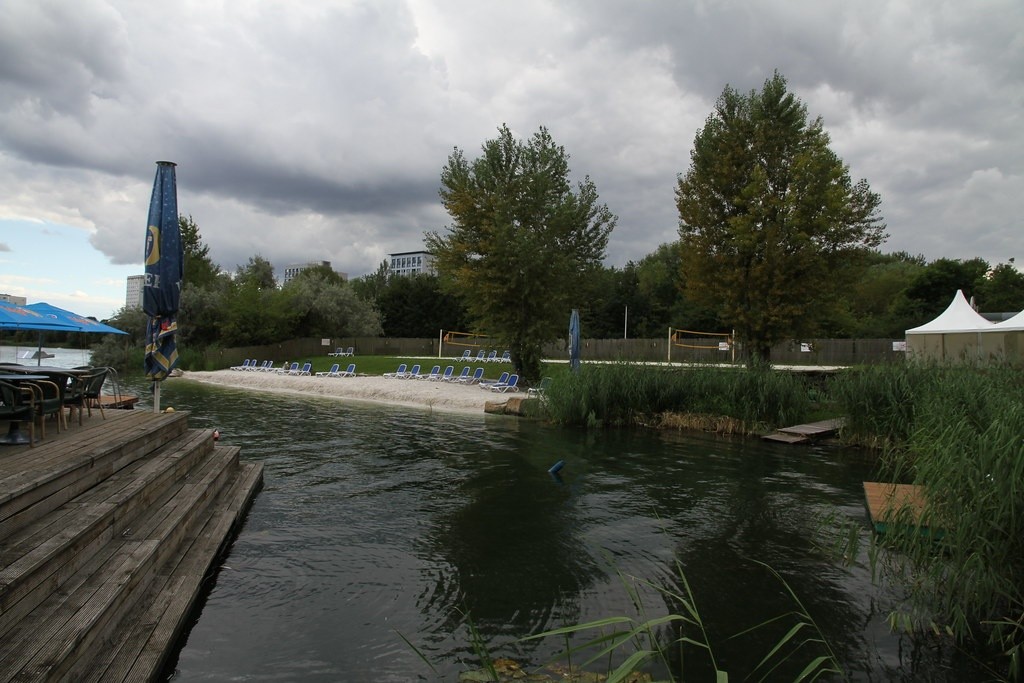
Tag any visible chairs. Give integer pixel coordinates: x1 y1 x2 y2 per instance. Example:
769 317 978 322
416 366 441 380
498 350 512 364
290 363 311 376
527 377 554 399
399 364 420 380
383 363 407 379
332 364 356 378
328 347 354 357
479 371 510 390
484 350 499 363
278 362 299 375
491 374 520 393
451 350 471 363
469 350 487 363
230 359 273 373
0 362 109 449
447 365 471 383
458 368 484 385
430 366 454 382
316 364 340 378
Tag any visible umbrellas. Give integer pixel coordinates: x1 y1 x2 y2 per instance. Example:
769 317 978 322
568 309 581 369
139 160 182 413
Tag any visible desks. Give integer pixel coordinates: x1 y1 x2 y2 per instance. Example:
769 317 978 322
0 374 50 445
0 366 90 426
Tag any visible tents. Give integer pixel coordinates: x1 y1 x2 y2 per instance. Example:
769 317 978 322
905 288 995 366
988 308 1024 366
